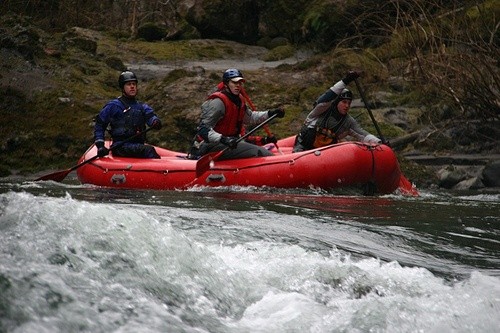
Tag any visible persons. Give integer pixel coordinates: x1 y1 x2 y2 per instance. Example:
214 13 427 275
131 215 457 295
292 71 389 153
94 71 162 158
190 68 285 160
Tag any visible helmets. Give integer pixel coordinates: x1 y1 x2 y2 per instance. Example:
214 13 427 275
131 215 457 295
338 87 353 99
119 71 137 88
223 69 244 85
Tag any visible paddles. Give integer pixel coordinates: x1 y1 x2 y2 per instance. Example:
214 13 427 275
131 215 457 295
36 125 154 182
352 73 419 197
196 113 276 178
241 88 283 155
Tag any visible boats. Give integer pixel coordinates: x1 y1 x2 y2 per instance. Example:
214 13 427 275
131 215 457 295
76 131 403 198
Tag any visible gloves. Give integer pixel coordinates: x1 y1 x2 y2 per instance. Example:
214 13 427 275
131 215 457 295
220 136 237 149
97 147 109 157
346 69 359 82
268 107 285 118
152 119 161 130
